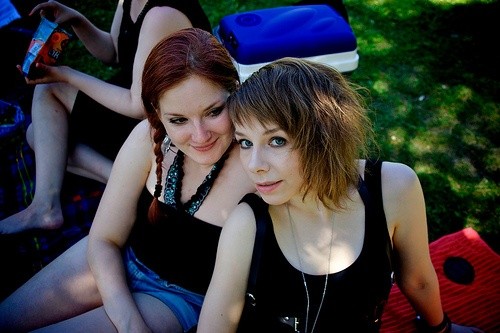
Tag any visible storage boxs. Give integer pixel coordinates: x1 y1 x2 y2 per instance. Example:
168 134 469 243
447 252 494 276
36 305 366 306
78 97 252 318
211 4 359 86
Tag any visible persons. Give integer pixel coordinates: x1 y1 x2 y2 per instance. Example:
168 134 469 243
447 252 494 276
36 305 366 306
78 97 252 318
0 28 257 333
0 0 213 235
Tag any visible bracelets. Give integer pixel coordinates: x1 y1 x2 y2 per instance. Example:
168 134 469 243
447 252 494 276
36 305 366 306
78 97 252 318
424 311 448 333
443 318 451 333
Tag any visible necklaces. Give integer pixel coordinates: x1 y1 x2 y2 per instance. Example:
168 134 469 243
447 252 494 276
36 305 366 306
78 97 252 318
196 56 482 333
282 200 338 333
